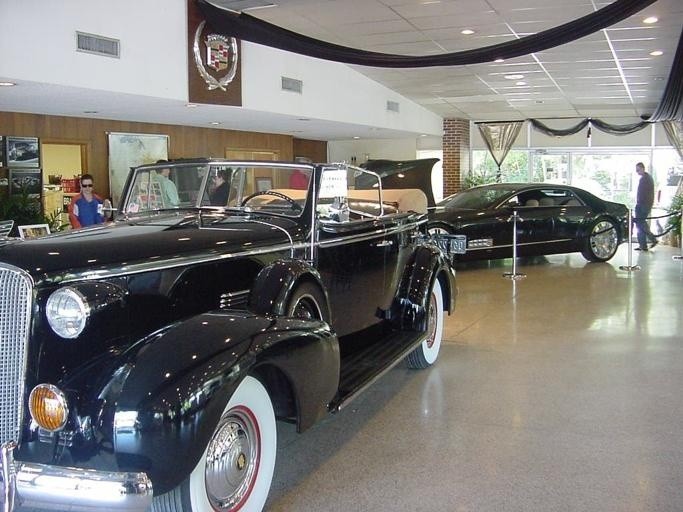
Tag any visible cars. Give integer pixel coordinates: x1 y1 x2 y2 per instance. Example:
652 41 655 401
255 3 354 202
8 143 39 162
0 154 459 510
416 180 635 266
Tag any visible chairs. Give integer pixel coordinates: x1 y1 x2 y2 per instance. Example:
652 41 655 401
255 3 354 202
540 196 553 205
525 196 539 206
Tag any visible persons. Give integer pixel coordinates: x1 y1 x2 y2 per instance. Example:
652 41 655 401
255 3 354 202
67 174 107 230
208 168 236 205
632 161 657 251
146 159 180 210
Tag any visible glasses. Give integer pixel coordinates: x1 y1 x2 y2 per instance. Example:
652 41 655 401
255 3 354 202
81 183 94 187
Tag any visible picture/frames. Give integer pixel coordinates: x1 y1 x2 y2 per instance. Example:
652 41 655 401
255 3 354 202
17 222 54 241
255 175 273 194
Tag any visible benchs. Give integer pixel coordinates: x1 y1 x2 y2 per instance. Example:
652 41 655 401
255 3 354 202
227 186 431 223
